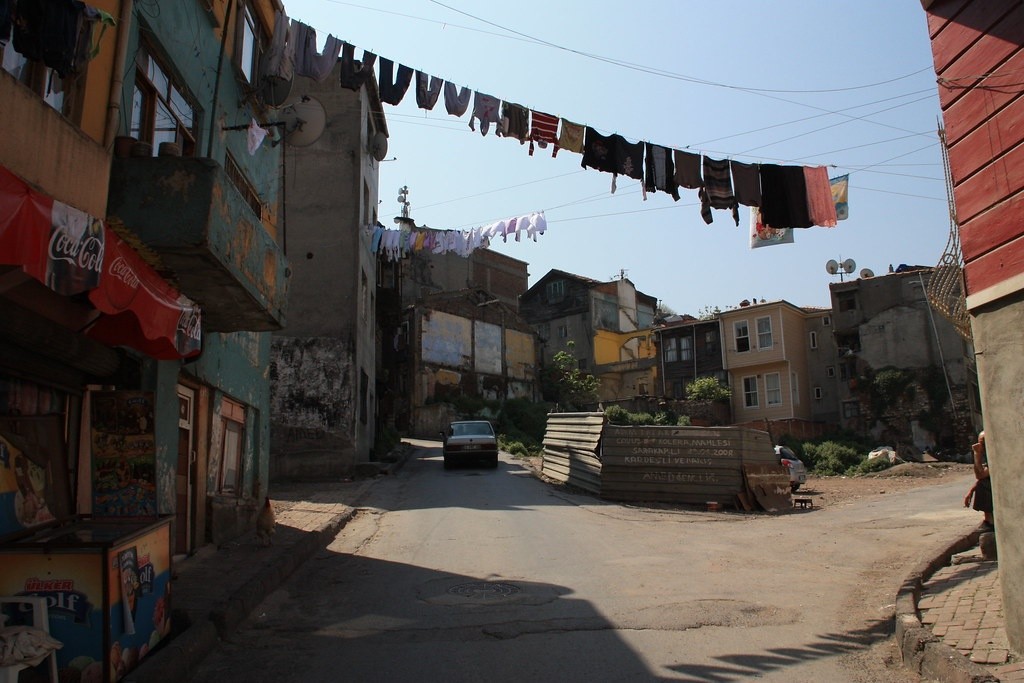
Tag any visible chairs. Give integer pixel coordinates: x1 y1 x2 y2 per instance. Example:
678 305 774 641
0 595 60 683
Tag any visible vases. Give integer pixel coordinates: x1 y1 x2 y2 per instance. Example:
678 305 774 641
553 340 602 412
114 136 179 159
681 375 731 426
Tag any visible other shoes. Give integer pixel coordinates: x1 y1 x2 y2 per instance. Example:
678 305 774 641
973 520 993 533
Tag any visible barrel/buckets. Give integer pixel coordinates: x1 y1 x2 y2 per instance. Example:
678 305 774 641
706 502 718 511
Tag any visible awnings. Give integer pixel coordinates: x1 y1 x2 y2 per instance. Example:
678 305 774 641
0 165 200 361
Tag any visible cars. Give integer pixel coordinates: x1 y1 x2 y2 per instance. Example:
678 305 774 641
439 421 499 470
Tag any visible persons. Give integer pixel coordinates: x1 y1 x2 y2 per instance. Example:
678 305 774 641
964 430 994 529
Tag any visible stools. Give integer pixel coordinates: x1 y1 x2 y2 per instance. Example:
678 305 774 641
795 499 812 509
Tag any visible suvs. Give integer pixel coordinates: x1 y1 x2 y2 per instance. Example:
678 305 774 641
774 446 806 491
